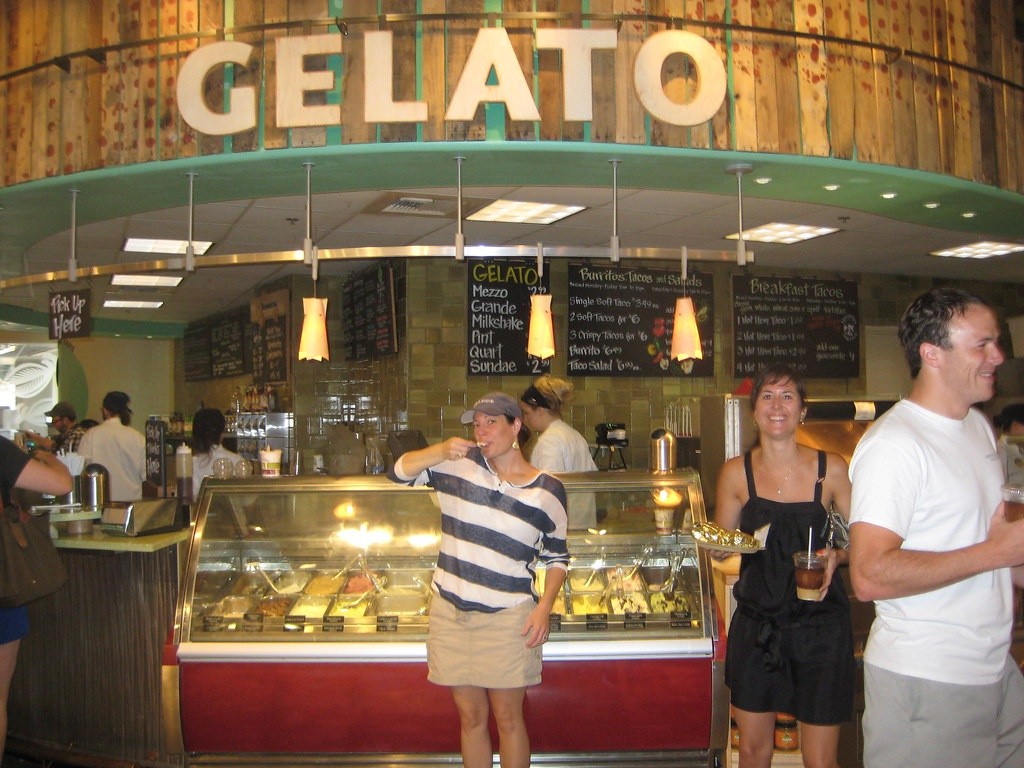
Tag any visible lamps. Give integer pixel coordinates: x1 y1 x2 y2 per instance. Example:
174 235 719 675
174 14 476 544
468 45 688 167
67 159 748 360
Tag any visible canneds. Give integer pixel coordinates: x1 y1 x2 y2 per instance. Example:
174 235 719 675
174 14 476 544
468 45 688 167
774 713 800 752
731 714 740 750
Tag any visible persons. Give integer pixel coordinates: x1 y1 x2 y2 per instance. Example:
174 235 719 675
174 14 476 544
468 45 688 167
172 409 245 496
387 391 570 767
0 434 73 764
22 401 97 453
520 376 598 532
78 391 147 501
847 289 1024 768
992 403 1024 486
707 367 853 768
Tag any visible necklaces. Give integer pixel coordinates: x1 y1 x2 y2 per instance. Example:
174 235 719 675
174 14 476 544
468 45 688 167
768 467 790 494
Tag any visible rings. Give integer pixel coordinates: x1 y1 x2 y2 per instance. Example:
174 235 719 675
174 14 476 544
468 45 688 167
722 555 726 559
458 455 463 458
543 637 546 639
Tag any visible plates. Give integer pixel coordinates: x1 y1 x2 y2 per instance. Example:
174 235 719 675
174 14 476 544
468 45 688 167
690 538 759 553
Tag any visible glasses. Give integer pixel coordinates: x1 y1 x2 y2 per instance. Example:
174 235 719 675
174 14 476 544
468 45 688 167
52 419 62 424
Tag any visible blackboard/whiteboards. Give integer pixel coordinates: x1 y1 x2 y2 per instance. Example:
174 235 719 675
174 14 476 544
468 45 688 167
145 423 163 485
731 275 860 380
251 321 289 383
341 267 395 360
566 264 714 379
48 289 90 339
467 259 550 377
181 314 245 379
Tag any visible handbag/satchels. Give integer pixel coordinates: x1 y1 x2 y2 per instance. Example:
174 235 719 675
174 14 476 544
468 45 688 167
820 510 850 552
0 489 72 609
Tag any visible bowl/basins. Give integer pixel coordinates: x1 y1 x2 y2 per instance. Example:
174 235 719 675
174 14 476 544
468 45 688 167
211 458 253 477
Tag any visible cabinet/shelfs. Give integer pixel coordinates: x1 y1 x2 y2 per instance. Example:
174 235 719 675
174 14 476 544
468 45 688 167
237 412 290 462
164 469 715 662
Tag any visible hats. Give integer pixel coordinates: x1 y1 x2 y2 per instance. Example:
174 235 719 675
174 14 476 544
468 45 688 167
44 401 77 418
460 391 523 425
102 391 132 413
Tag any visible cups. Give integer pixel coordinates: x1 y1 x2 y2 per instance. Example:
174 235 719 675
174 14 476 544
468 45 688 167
259 451 281 478
793 550 828 601
1000 483 1024 522
56 475 81 504
653 506 674 535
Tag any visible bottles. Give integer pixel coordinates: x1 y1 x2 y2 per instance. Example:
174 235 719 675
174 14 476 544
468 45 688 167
40 436 56 505
231 384 277 412
161 412 193 437
224 416 237 433
176 442 193 498
